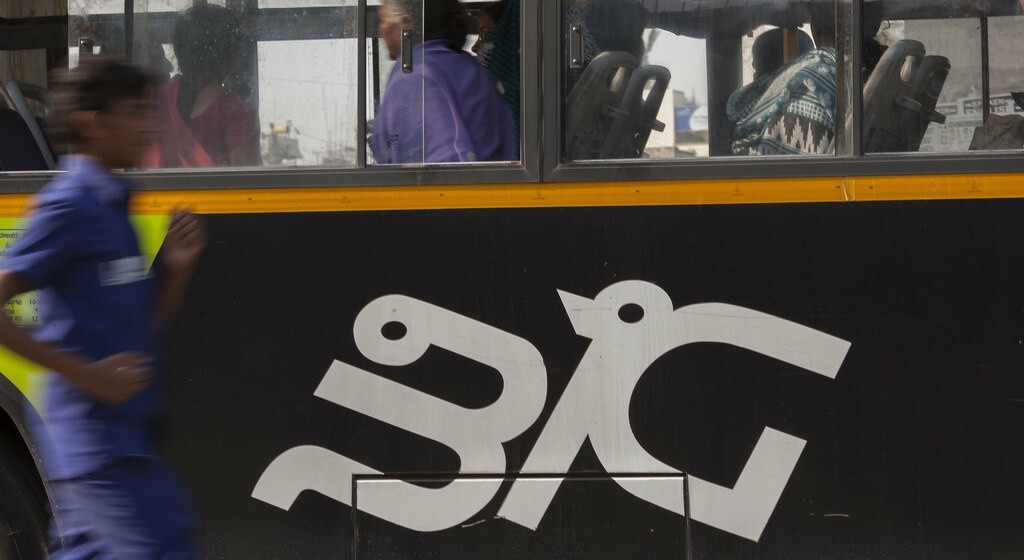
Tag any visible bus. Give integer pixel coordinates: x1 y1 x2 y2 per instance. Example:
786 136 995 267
2 0 1024 559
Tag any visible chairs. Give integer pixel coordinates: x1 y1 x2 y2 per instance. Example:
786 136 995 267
825 39 952 155
565 51 670 161
2 78 59 169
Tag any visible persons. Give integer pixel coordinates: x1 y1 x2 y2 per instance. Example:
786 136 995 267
0 56 207 560
135 6 260 169
368 0 517 163
724 0 890 159
